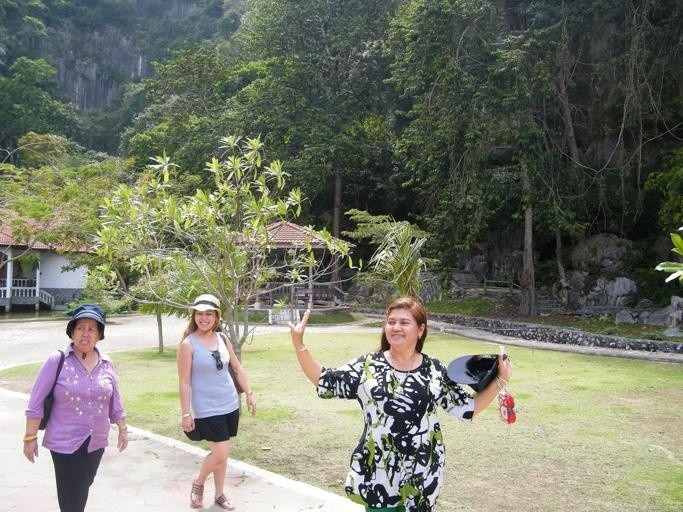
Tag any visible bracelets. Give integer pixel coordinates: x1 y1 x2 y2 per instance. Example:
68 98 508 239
296 346 308 353
246 389 252 395
182 413 191 419
23 435 37 443
496 377 503 391
120 426 127 430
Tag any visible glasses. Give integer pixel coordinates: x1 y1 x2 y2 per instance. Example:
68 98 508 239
210 349 224 371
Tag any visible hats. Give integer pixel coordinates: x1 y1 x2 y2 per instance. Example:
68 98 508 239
188 291 222 317
443 350 508 394
64 303 107 341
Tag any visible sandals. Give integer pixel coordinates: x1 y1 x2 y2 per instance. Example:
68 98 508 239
188 477 205 509
212 492 236 511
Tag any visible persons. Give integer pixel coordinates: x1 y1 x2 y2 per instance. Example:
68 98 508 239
23 304 128 512
176 294 257 511
287 296 513 512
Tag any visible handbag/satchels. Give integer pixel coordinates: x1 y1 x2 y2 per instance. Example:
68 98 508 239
35 346 68 432
215 331 246 393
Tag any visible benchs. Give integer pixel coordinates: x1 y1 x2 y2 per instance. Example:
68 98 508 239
292 285 333 306
244 287 273 306
